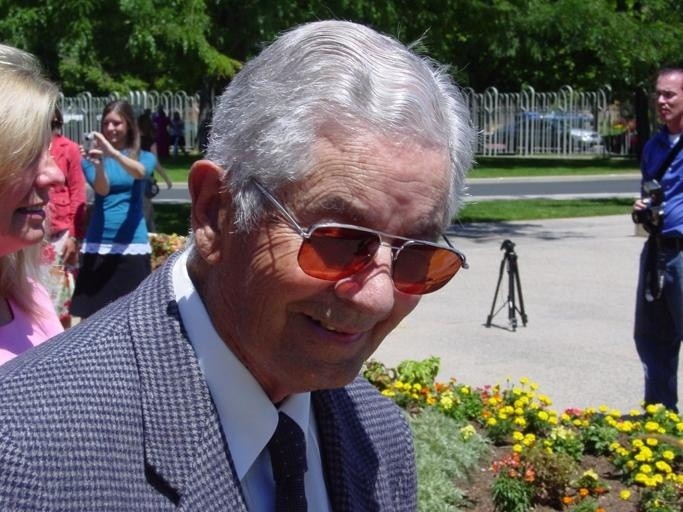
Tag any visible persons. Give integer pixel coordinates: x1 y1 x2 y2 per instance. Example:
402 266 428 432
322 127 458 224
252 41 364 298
0 43 64 370
632 62 681 417
135 103 212 164
135 113 173 235
71 99 155 318
0 14 478 510
36 104 86 329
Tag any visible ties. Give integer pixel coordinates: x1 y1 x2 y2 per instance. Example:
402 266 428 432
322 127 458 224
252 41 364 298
266 412 307 512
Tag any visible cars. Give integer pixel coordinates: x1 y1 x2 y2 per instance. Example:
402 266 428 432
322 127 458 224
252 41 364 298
479 108 612 159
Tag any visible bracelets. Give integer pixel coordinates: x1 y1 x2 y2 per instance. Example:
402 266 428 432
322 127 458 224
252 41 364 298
70 236 78 244
111 150 120 160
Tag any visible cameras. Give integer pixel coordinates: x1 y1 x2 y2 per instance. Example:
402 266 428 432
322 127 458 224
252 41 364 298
632 179 665 234
82 132 95 151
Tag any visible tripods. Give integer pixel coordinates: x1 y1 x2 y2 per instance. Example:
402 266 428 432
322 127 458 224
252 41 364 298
487 240 527 330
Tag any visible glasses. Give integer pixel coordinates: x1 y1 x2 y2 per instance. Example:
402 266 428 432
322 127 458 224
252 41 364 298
251 178 468 294
